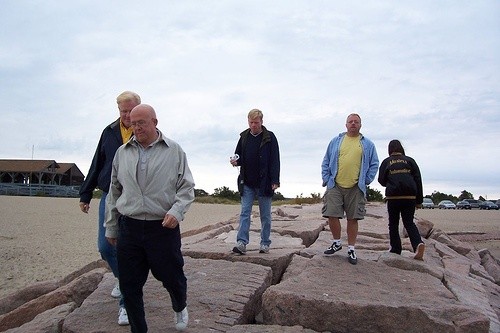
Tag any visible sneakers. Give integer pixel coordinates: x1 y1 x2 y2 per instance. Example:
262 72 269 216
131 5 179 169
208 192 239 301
175 306 188 330
347 249 357 265
259 244 270 253
232 240 247 255
112 281 121 297
324 241 342 256
116 308 129 325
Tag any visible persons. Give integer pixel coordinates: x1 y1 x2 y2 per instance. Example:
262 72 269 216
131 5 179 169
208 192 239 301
103 103 195 333
230 109 281 255
377 140 425 261
78 90 142 326
321 113 379 264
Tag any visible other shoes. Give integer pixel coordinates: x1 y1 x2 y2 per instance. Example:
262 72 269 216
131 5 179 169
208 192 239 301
414 243 425 258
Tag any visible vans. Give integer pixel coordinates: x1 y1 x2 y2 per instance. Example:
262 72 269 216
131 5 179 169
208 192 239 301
463 199 483 208
417 198 434 209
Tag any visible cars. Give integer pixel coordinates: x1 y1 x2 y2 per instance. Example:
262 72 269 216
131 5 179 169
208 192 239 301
438 200 456 210
457 201 472 210
479 199 500 210
